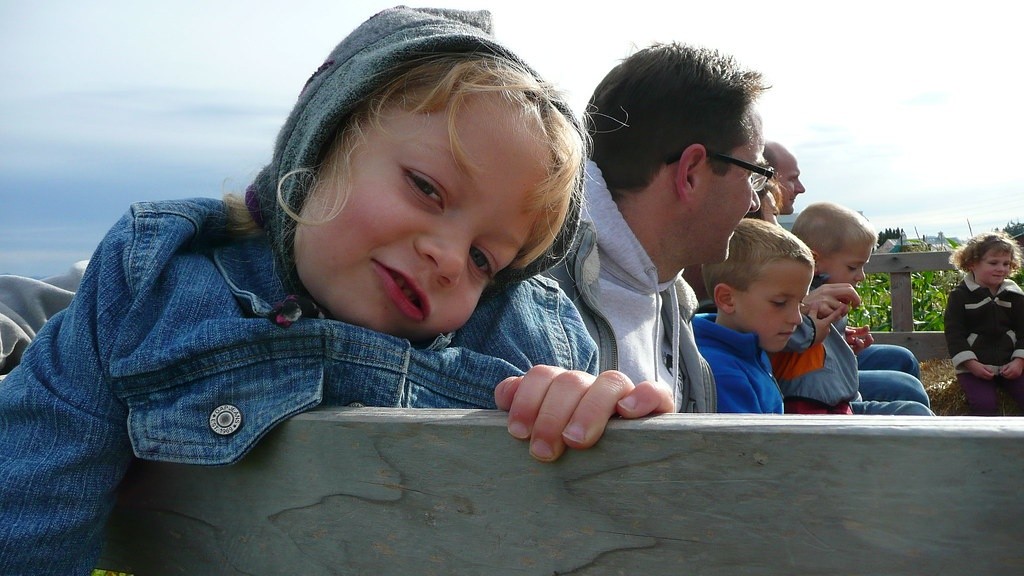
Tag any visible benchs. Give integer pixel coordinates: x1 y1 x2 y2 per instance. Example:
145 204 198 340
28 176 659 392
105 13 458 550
88 403 1024 576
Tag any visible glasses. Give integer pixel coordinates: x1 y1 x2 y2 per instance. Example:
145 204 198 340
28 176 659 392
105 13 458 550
660 149 773 191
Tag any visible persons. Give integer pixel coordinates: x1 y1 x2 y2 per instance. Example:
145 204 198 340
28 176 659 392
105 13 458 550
0 4 1023 576
